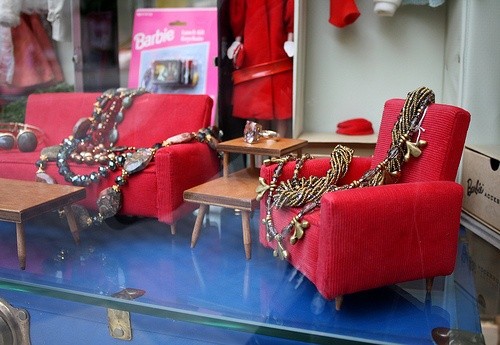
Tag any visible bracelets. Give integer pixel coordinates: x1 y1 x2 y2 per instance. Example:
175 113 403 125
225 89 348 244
0 120 45 152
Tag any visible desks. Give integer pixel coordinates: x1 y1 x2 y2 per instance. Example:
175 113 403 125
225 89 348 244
215 134 308 181
0 176 87 271
183 165 265 262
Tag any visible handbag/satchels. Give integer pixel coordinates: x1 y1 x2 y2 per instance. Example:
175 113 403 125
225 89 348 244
226 41 245 69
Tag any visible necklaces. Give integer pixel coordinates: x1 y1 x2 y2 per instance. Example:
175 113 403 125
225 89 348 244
255 86 436 261
36 88 227 229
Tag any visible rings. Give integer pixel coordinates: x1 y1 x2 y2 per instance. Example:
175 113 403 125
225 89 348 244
243 119 286 144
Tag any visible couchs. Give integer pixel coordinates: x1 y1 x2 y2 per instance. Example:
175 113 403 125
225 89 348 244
0 93 220 236
258 98 472 312
